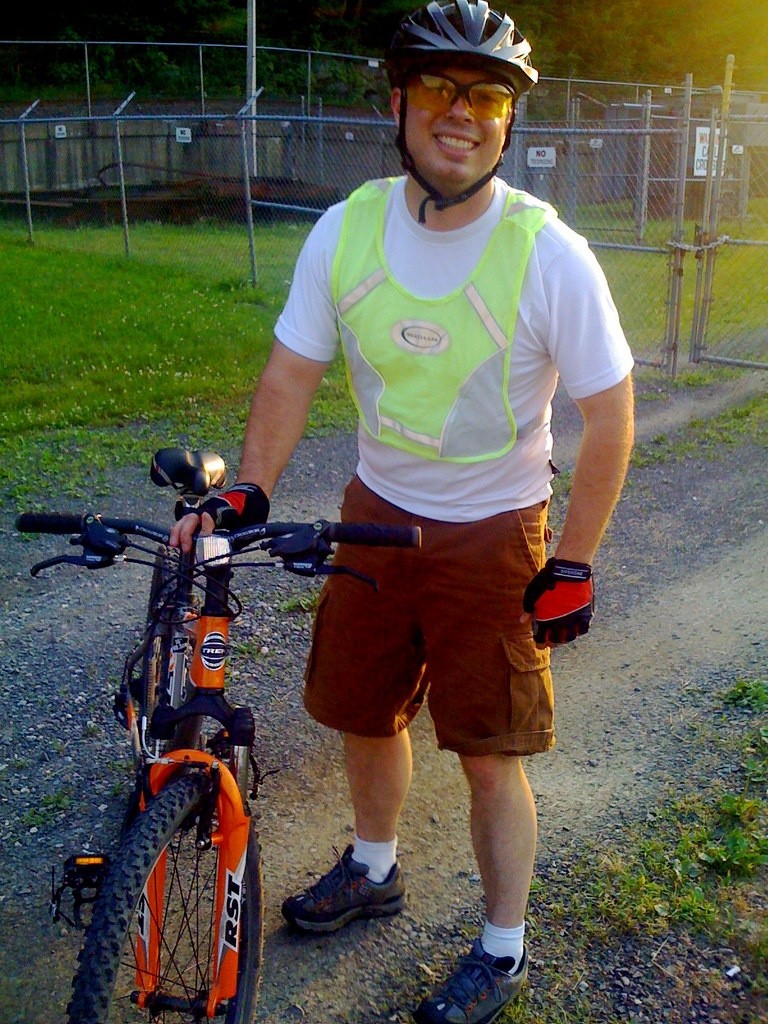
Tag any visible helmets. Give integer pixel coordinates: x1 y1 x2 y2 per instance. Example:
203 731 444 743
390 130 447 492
386 0 539 99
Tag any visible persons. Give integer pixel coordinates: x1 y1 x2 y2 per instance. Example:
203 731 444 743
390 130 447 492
171 0 635 1024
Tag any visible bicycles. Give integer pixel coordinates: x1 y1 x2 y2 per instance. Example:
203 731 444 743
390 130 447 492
16 443 424 1023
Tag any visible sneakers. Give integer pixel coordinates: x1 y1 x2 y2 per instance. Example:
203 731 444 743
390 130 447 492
419 937 529 1024
281 844 404 932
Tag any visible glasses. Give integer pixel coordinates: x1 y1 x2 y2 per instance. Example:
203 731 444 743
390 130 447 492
403 72 515 120
